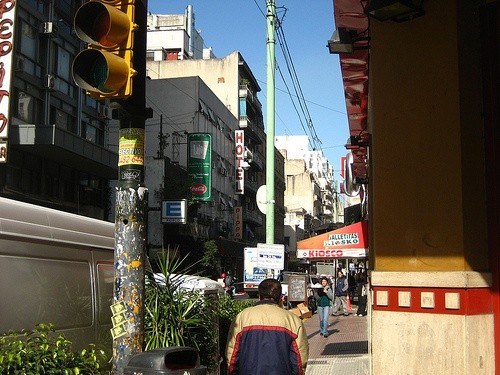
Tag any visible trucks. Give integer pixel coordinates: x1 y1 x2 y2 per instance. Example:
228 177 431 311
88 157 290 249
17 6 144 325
243 247 281 297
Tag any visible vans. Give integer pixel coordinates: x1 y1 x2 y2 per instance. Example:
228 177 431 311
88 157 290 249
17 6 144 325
144 272 225 306
0 196 175 375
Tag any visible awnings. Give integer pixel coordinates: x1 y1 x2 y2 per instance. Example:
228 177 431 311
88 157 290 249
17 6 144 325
296 221 369 258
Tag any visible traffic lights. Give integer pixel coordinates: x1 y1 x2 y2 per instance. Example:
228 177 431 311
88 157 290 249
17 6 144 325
70 0 139 100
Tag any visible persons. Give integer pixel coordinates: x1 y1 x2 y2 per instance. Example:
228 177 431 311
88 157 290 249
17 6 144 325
225 278 309 375
219 268 368 337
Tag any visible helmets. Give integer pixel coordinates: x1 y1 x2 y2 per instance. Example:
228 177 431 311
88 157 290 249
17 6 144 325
227 270 232 274
221 273 226 277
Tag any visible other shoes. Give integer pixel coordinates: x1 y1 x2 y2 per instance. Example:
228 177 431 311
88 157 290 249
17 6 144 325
320 333 323 336
324 335 328 337
